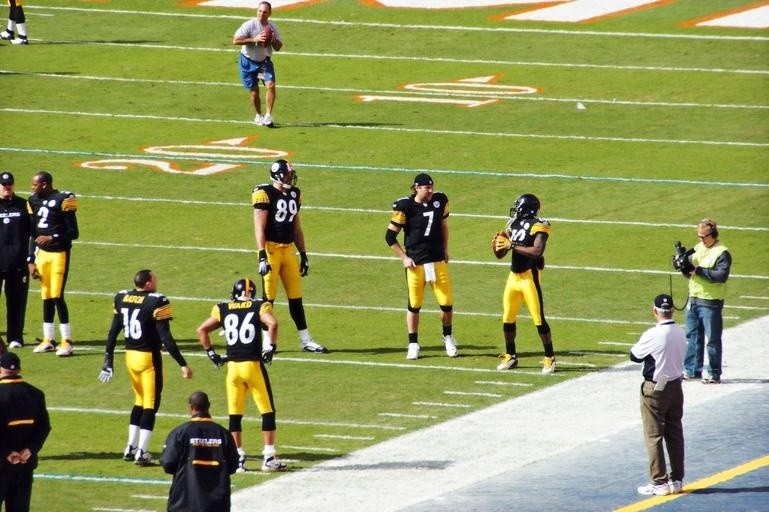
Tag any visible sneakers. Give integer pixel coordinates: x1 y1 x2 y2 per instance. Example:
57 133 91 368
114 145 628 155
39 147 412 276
0 30 29 44
303 344 328 354
638 480 683 495
124 445 156 466
408 344 420 360
255 114 274 128
683 372 722 385
234 459 248 473
262 456 286 472
441 335 458 356
8 337 73 357
498 353 518 371
542 356 556 374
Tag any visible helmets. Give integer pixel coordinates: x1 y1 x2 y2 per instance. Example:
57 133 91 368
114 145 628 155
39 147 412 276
232 278 256 300
510 194 540 217
271 160 295 190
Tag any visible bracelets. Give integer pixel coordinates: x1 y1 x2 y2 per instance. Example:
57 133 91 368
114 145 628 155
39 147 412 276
206 347 216 358
511 243 515 249
258 248 267 262
299 252 307 260
268 344 278 353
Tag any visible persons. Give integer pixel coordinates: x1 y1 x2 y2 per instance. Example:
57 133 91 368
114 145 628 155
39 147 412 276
493 193 555 375
0 172 30 349
675 217 732 383
195 278 288 472
630 294 686 496
27 171 79 356
159 392 240 512
233 1 282 126
0 352 52 512
0 0 28 45
251 160 329 354
98 270 192 466
385 174 458 360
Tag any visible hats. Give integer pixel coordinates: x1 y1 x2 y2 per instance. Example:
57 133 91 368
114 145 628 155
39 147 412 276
1 353 20 371
0 172 14 185
656 295 674 310
409 174 432 191
698 219 715 237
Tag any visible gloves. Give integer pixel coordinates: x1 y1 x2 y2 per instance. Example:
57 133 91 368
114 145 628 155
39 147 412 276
497 236 516 249
262 344 278 365
99 362 113 383
258 249 272 276
684 263 699 275
206 346 226 369
300 253 309 278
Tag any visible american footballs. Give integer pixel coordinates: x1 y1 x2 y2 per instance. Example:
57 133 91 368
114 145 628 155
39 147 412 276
492 232 511 259
260 25 274 50
